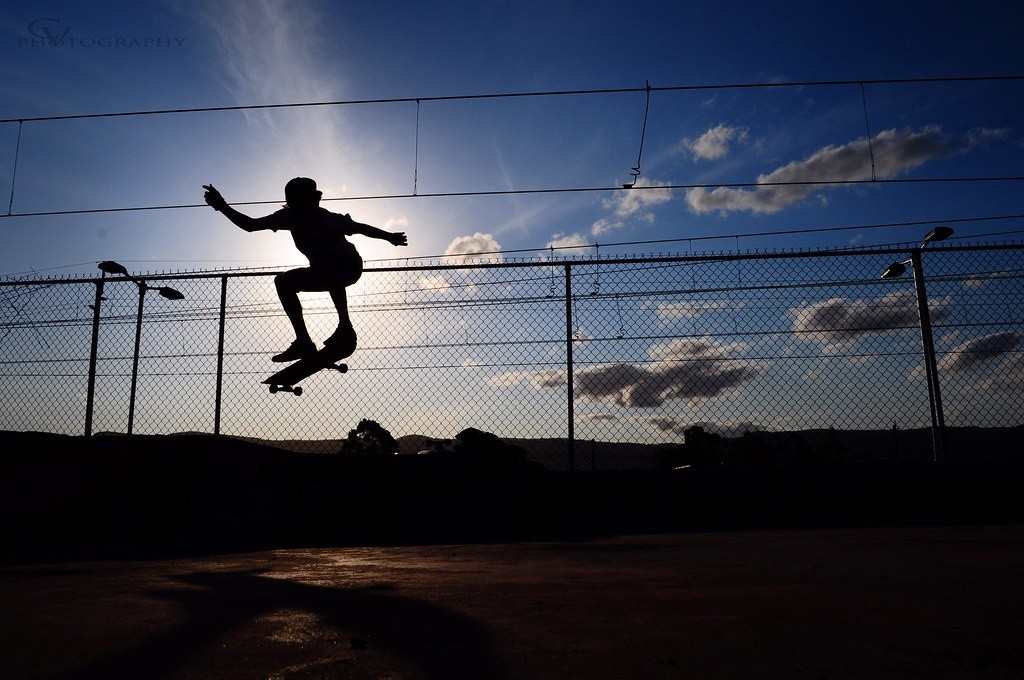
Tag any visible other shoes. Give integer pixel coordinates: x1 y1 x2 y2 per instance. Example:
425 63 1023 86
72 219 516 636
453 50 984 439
272 338 317 362
323 324 357 346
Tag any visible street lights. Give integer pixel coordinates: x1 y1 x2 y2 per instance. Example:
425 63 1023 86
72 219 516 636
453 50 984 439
878 226 956 461
96 259 185 434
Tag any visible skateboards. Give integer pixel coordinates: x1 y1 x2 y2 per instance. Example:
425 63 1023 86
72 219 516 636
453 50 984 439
261 339 357 396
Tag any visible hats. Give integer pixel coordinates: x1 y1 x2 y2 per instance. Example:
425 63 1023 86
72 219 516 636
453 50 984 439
284 177 322 197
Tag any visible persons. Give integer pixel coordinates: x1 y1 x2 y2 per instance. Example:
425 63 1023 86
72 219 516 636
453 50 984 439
203 175 408 362
